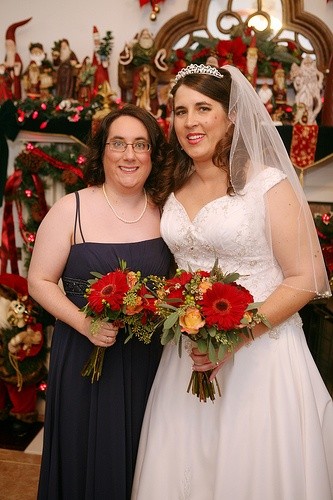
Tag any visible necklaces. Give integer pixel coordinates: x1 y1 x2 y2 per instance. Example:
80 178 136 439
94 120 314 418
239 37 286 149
102 183 147 223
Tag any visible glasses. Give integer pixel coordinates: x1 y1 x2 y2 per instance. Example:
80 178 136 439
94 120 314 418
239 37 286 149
105 140 151 153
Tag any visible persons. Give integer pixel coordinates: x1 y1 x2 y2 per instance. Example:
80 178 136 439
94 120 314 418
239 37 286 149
27 104 178 500
130 60 333 500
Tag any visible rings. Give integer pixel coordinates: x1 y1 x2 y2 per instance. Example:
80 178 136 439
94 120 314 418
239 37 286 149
216 360 219 367
105 336 108 343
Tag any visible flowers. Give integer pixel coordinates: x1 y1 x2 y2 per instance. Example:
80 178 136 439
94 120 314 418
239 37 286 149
78 261 163 381
145 258 270 404
